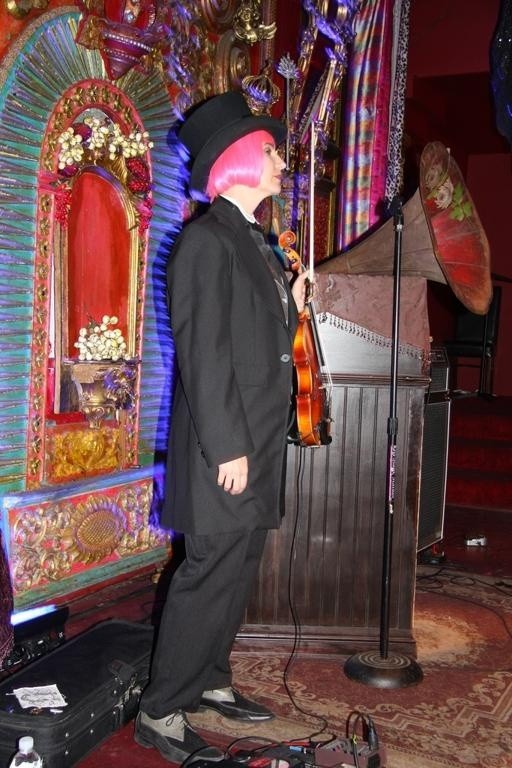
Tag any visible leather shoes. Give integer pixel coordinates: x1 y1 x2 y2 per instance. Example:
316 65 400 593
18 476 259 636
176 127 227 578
134 709 225 764
200 687 275 722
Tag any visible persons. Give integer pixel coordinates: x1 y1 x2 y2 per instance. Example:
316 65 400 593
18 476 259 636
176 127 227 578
132 92 322 768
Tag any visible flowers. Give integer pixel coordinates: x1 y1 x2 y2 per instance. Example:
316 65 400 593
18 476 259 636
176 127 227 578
67 305 132 363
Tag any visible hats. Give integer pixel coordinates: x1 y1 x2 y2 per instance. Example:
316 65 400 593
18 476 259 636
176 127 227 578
179 93 288 192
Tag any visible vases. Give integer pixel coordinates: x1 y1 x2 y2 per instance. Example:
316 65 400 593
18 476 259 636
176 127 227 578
66 360 137 427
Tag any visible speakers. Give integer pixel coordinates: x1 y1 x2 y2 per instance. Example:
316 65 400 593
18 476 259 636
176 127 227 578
412 341 456 552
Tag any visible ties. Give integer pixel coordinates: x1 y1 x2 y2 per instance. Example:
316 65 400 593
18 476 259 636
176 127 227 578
249 229 288 327
